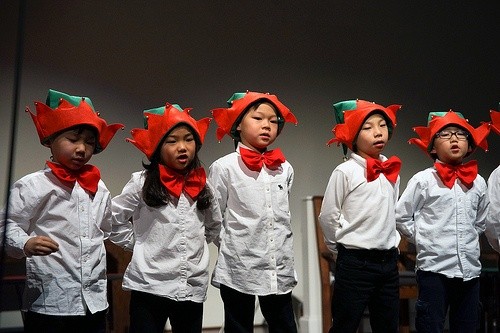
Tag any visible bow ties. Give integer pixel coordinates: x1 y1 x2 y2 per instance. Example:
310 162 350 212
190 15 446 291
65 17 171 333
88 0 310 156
46 160 100 194
366 156 402 183
434 160 478 188
158 164 205 199
237 146 286 172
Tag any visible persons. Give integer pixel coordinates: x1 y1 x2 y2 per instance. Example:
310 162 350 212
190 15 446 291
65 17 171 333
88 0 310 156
396 108 490 333
111 101 222 333
318 97 403 333
484 109 500 252
207 90 298 333
1 88 114 333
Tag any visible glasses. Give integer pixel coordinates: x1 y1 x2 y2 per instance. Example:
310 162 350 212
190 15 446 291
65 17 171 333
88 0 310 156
435 132 470 139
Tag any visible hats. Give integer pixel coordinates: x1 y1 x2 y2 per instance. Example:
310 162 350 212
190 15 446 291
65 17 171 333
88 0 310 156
408 109 492 160
210 90 299 143
325 99 402 162
125 103 213 161
24 89 125 154
479 108 500 135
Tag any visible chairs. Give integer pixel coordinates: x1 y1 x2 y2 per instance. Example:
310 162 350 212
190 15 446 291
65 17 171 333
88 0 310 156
311 196 420 333
